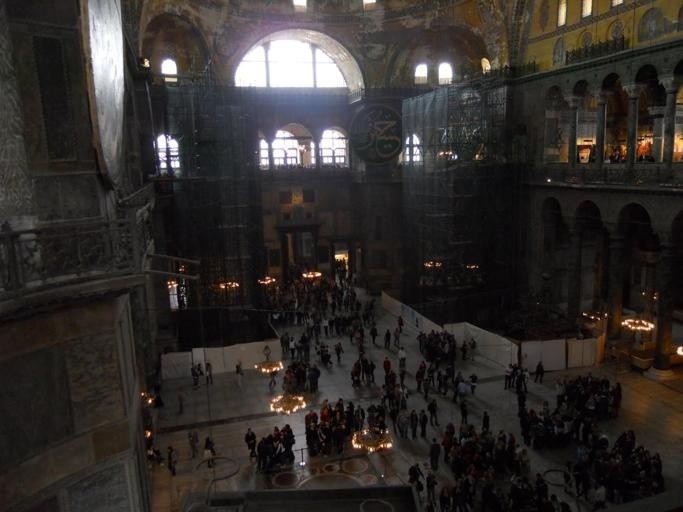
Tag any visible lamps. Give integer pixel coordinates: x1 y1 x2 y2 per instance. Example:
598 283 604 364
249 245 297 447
582 309 609 323
144 429 151 439
252 353 285 375
267 350 308 415
621 274 658 332
352 333 395 452
166 255 483 295
641 286 658 302
430 142 554 186
142 392 156 407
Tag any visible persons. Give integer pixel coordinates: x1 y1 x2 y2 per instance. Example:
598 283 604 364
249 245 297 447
190 359 216 386
204 434 218 468
165 448 178 476
237 360 246 391
175 383 186 415
244 271 663 512
190 432 198 459
